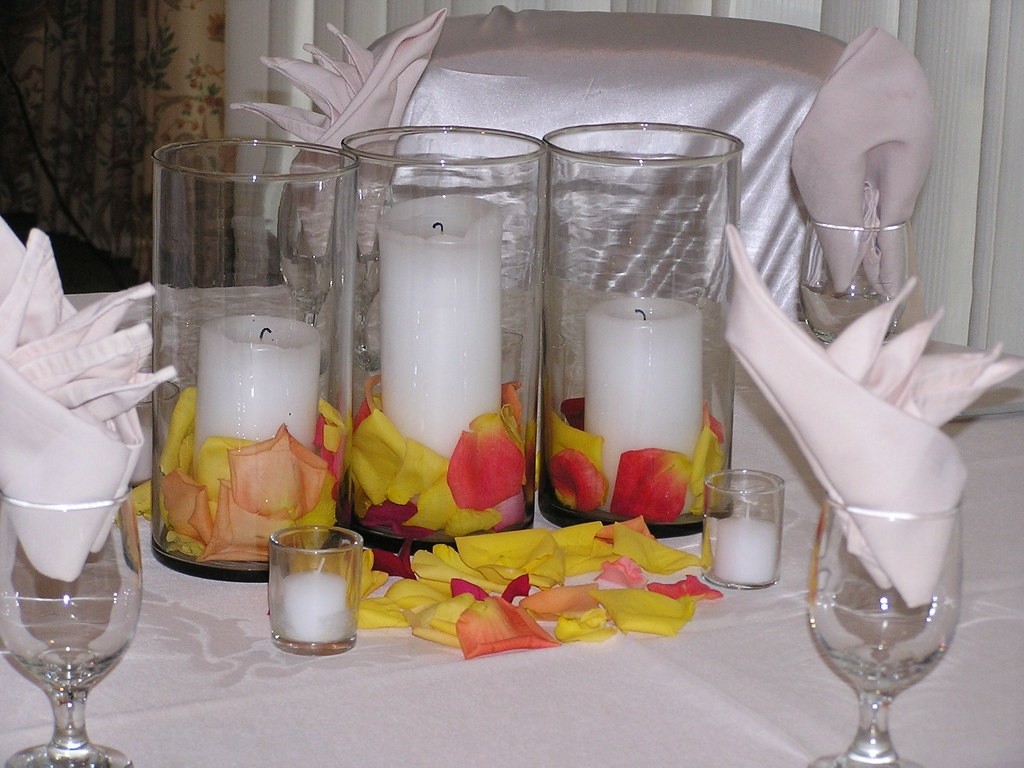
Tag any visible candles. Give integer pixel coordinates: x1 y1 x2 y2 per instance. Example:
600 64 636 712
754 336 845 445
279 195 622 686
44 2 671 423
192 314 320 457
279 557 350 644
377 194 503 458
713 505 778 584
584 296 706 516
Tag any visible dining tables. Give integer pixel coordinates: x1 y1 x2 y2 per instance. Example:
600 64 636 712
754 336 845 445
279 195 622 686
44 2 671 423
1 287 1023 768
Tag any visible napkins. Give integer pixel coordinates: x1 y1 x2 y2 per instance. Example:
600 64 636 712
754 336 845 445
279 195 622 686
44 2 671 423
227 2 444 265
0 217 179 588
719 222 1023 608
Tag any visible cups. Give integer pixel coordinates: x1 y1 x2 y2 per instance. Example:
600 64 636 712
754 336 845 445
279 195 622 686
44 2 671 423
543 117 741 535
338 126 544 543
278 138 391 364
266 519 364 655
797 213 910 348
141 135 348 578
700 468 785 588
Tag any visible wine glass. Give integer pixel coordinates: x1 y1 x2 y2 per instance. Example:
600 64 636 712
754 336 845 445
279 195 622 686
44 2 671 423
0 492 146 767
807 490 964 766
